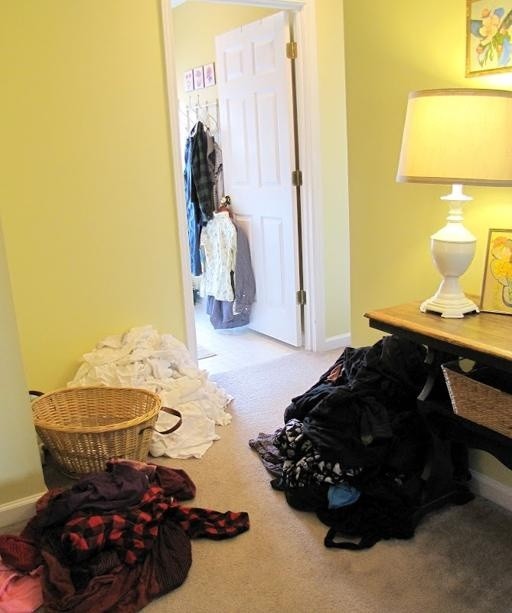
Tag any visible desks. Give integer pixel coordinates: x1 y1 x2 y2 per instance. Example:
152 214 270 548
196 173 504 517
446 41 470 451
360 293 512 510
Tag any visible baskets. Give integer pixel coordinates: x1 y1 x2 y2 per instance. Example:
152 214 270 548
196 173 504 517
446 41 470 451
29 386 183 480
441 356 512 440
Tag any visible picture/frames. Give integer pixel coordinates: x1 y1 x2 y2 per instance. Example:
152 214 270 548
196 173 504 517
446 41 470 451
463 0 512 80
479 228 512 315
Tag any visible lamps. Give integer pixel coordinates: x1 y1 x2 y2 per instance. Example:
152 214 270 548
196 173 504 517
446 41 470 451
394 83 512 320
